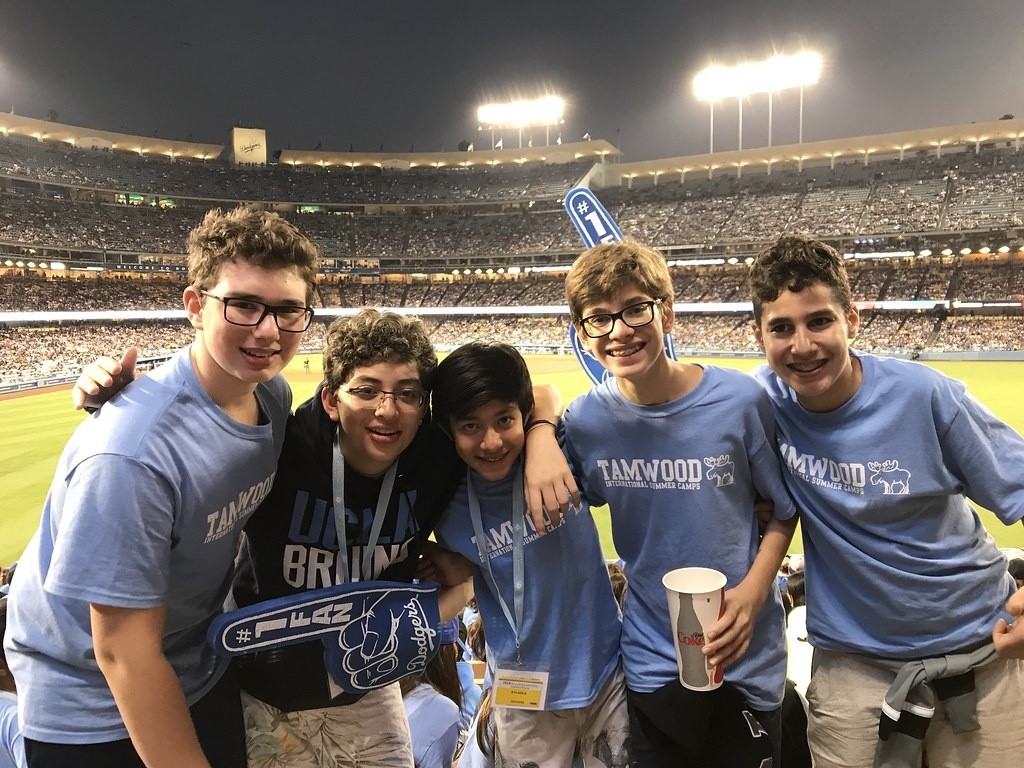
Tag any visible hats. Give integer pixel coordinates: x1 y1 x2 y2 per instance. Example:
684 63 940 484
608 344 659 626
439 616 460 644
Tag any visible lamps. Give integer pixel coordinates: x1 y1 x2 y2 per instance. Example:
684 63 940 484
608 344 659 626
458 141 474 151
582 132 592 142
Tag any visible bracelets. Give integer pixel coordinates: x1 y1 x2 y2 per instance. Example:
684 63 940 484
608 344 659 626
524 419 557 435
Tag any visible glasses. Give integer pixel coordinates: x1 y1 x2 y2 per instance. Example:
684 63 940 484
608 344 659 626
579 298 663 338
339 385 429 410
199 288 314 333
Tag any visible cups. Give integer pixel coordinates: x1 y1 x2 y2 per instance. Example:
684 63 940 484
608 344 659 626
663 567 727 691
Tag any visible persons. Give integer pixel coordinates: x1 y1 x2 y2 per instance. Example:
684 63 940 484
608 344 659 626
748 236 1023 767
3 203 796 767
993 587 1023 663
